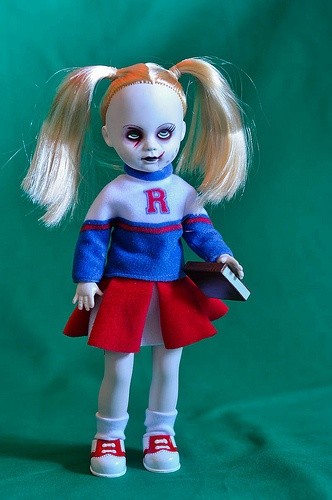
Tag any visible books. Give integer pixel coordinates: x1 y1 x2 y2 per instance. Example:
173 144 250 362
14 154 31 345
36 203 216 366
182 261 250 302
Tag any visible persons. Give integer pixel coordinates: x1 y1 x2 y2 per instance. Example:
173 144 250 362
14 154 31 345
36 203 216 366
18 56 251 481
